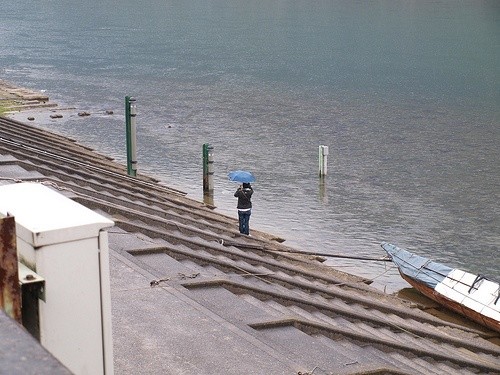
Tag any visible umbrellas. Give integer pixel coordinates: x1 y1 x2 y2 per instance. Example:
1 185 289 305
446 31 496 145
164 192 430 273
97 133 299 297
227 170 256 184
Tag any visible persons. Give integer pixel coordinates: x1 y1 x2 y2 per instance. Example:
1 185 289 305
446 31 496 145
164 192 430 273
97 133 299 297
234 182 254 236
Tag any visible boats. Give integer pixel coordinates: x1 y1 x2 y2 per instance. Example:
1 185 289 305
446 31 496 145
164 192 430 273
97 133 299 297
379 241 500 334
